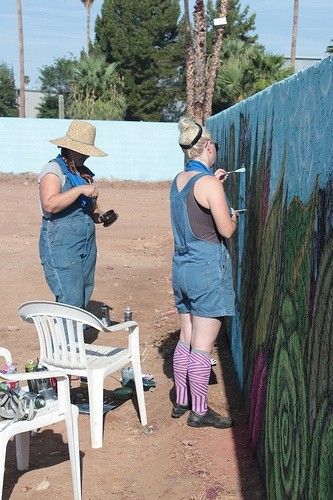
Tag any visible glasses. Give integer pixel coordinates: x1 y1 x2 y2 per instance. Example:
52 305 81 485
205 141 219 152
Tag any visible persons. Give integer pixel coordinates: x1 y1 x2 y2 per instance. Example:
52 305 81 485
171 117 241 430
36 120 119 380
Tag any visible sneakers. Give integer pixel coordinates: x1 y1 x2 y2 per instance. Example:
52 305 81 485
172 401 189 417
187 406 234 428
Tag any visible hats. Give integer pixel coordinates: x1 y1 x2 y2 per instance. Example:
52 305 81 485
49 120 108 156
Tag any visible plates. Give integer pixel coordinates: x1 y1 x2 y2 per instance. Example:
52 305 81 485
235 210 246 213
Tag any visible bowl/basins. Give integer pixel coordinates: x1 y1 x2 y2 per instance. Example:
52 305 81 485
112 387 133 399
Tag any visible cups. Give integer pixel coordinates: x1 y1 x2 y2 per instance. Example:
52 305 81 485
121 368 134 386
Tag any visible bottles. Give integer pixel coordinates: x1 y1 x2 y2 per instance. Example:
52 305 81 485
125 308 133 322
100 303 110 327
0 359 56 410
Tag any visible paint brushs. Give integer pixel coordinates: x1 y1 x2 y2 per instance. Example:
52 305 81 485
225 167 246 174
89 179 107 217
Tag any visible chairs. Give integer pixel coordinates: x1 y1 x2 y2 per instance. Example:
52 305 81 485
0 346 82 500
17 300 147 449
